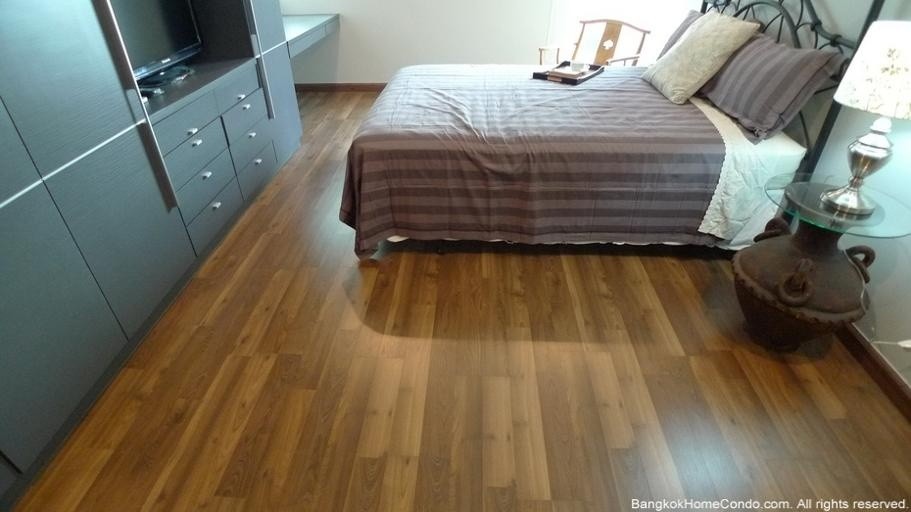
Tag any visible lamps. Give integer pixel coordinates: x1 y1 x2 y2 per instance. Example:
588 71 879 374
819 21 910 212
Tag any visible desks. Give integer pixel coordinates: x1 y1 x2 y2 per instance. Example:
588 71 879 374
281 14 340 58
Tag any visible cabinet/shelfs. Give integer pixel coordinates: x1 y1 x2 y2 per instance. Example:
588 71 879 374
0 0 305 512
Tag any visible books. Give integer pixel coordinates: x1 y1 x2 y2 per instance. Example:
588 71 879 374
548 64 590 78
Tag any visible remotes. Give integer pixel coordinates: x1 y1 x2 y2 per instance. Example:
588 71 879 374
139 86 163 97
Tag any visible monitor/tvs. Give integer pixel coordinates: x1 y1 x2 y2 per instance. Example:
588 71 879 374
110 0 208 88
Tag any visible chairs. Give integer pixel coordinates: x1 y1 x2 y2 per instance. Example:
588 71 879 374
538 19 651 66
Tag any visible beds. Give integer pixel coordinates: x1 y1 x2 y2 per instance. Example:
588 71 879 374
339 0 887 259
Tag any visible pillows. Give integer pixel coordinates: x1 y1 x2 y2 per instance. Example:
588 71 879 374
641 10 850 145
